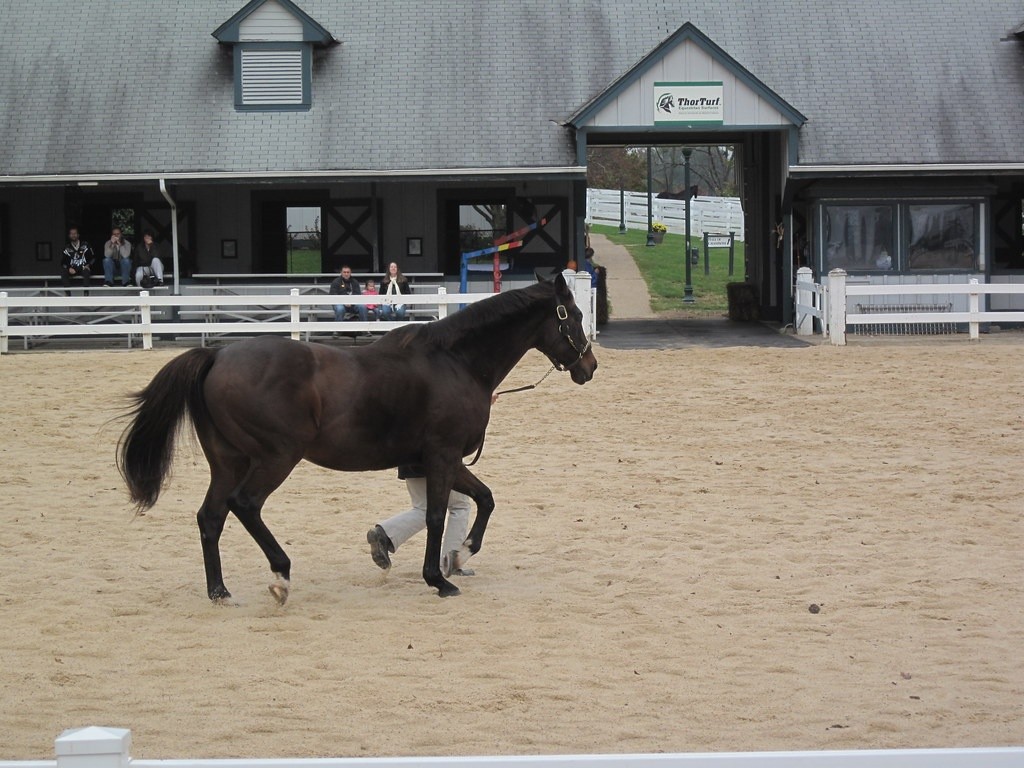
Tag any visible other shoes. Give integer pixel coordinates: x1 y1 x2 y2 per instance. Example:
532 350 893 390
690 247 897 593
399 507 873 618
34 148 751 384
596 331 600 335
455 568 475 577
333 333 338 339
366 527 392 570
103 282 112 287
159 281 165 285
125 283 133 286
366 333 372 336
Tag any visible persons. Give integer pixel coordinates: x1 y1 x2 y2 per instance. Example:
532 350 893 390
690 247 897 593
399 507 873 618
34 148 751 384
550 247 595 274
101 227 134 287
134 229 165 287
60 226 96 296
379 261 412 321
361 279 381 321
330 266 372 338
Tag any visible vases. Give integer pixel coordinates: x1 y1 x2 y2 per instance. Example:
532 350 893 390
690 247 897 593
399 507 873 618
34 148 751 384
653 232 664 244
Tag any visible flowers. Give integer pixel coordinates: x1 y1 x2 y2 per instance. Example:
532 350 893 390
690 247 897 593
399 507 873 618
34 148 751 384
653 222 667 231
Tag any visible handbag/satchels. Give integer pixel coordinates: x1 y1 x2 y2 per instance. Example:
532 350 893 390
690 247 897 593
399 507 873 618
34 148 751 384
141 266 159 288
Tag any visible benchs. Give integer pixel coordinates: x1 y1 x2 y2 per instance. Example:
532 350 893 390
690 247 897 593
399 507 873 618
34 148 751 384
0 274 171 347
175 273 443 347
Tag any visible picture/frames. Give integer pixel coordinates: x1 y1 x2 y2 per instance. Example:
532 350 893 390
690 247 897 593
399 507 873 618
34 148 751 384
221 239 237 258
36 243 52 261
407 238 424 258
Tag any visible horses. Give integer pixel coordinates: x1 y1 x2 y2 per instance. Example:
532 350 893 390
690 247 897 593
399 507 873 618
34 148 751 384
101 272 598 605
